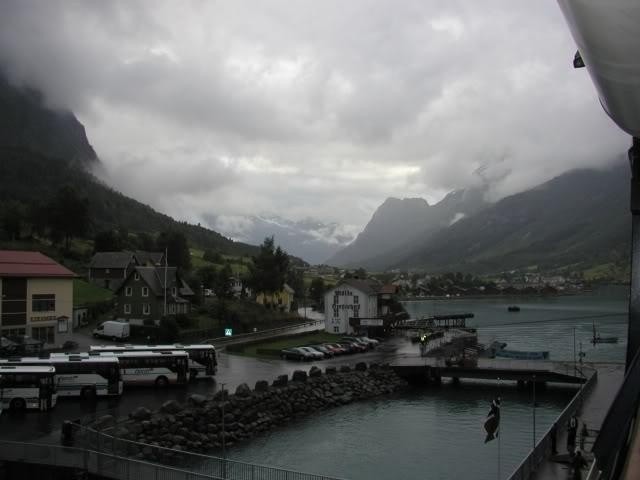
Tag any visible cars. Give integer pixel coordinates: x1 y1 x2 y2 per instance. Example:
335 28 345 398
411 328 444 344
280 336 379 361
204 289 216 297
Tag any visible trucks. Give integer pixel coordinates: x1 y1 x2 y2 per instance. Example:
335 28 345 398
92 321 130 342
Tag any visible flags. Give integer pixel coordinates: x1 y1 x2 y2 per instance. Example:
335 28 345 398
483 397 500 443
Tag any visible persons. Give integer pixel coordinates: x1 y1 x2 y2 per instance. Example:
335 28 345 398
550 415 589 480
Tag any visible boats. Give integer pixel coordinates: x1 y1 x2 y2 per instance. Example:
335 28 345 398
508 306 521 311
591 319 619 344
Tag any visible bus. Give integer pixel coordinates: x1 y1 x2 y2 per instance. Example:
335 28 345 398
0 356 123 400
89 343 217 383
0 366 58 413
50 350 190 388
